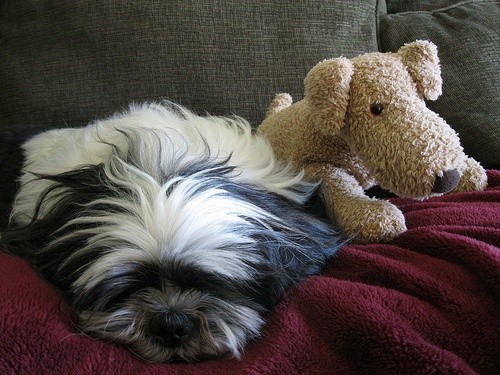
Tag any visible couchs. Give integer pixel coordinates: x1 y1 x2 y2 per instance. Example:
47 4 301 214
0 0 500 375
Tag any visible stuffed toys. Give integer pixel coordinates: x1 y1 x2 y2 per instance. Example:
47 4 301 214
256 41 488 246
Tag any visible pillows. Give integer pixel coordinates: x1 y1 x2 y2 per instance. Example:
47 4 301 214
376 0 500 171
0 0 375 148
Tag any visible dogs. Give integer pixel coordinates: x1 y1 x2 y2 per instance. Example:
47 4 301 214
5 99 362 364
